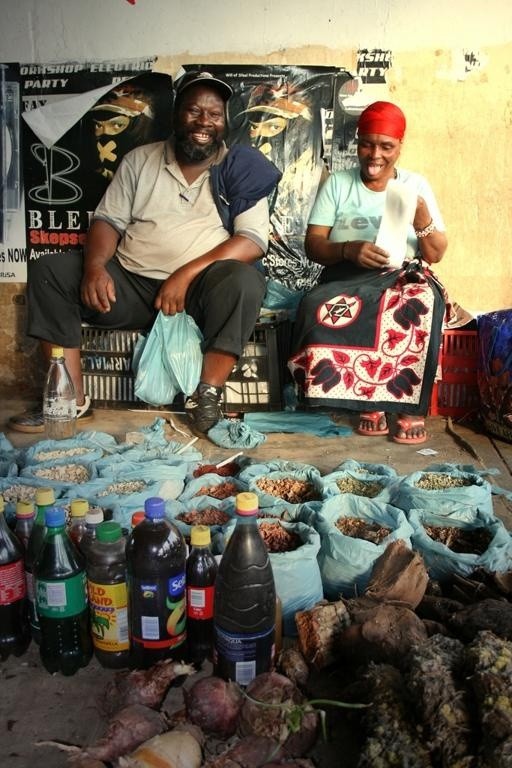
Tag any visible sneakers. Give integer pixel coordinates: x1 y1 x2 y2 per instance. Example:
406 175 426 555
8 393 94 433
183 383 223 439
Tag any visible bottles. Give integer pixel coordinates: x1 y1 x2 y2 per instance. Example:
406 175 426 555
41 345 78 440
213 491 275 683
126 495 188 670
68 497 129 671
130 511 147 537
0 487 94 677
181 525 218 668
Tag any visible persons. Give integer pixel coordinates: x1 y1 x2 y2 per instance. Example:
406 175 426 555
302 101 449 445
231 81 320 202
23 73 282 438
80 83 157 183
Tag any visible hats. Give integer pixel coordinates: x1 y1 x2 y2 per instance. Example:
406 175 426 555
358 100 406 139
89 87 159 122
176 69 234 102
230 81 318 124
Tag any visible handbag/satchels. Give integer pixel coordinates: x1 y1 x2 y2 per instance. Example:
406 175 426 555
477 307 512 378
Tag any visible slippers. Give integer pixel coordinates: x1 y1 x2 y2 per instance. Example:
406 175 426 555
391 416 427 444
357 409 390 435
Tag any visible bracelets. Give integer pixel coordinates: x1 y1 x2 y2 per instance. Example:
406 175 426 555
414 220 436 238
340 238 352 263
